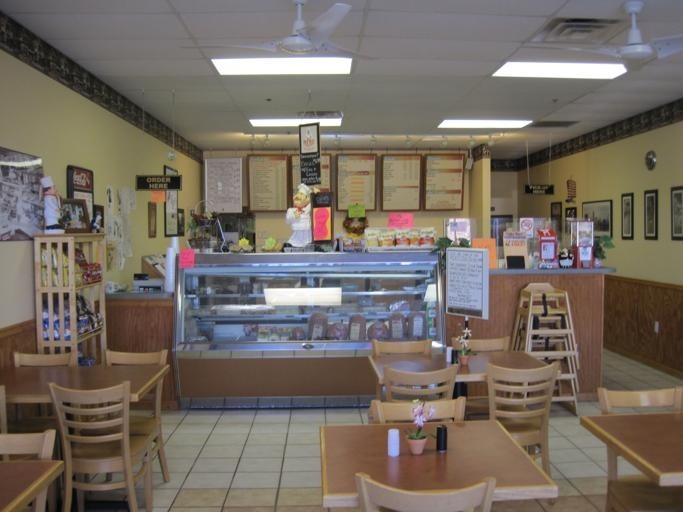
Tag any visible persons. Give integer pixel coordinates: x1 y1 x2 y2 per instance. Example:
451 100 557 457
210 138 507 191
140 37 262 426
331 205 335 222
39 175 66 234
280 180 324 251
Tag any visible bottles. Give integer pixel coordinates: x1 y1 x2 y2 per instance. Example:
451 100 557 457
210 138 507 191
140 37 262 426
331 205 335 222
436 424 448 452
446 347 452 362
387 427 399 457
451 349 457 364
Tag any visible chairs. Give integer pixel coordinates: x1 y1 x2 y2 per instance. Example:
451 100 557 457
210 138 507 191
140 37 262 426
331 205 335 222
316 281 683 511
0 346 171 511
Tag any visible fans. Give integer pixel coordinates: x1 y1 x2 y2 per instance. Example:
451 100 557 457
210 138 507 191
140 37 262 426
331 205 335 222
509 1 683 63
177 0 383 62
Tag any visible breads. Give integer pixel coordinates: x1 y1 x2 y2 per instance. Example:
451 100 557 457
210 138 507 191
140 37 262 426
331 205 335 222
292 312 427 341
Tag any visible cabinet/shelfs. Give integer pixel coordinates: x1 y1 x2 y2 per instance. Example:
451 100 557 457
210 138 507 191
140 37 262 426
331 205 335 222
32 230 109 369
172 246 445 411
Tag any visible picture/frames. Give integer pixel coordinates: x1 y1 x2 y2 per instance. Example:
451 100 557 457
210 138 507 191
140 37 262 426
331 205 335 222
60 197 91 234
550 186 682 242
